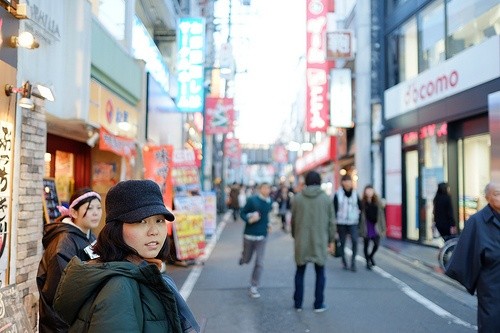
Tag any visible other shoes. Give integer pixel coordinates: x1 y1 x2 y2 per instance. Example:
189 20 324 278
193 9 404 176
315 304 328 313
367 254 375 268
249 286 260 298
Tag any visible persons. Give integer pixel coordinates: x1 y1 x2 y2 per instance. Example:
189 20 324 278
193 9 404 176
360 184 385 270
333 174 362 272
225 181 297 229
36 187 103 333
54 179 201 333
291 171 338 312
445 178 500 333
433 183 457 267
238 182 273 298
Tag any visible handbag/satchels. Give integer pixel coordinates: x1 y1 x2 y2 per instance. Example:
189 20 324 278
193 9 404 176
330 236 341 258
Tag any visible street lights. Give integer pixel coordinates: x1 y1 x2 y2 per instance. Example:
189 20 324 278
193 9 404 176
200 65 232 193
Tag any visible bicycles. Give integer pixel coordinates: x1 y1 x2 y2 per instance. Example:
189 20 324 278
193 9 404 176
438 235 459 273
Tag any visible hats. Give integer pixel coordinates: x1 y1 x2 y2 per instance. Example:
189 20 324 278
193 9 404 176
105 180 175 224
